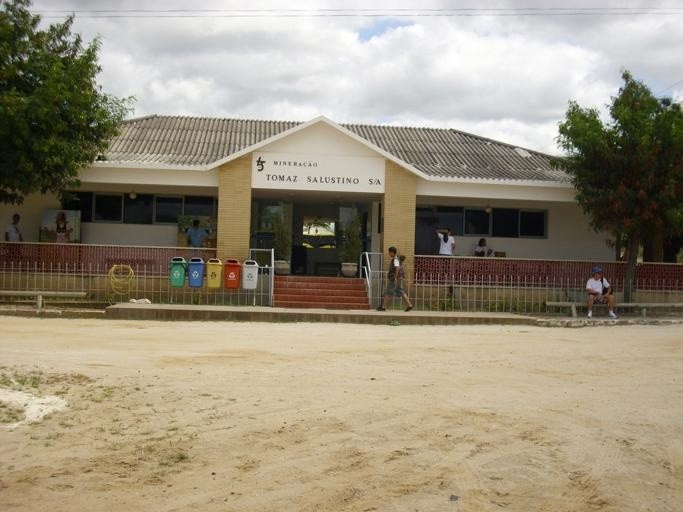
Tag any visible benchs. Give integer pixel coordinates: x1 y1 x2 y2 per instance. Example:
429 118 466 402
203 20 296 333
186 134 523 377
545 300 683 318
0 290 87 309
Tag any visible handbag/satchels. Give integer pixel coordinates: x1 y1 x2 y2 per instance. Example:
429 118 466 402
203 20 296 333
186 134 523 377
602 287 613 295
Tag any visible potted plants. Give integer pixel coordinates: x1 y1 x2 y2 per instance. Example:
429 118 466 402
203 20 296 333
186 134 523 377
272 220 293 276
338 225 364 278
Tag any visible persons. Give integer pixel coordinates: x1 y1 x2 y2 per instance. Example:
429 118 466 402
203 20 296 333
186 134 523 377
435 227 454 256
586 266 616 318
398 255 406 280
4 212 23 243
186 219 207 248
374 246 417 314
474 237 494 257
53 211 68 244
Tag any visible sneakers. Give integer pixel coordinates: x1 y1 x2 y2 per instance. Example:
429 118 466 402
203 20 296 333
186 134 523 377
608 313 616 317
405 307 412 311
588 312 593 318
377 307 385 311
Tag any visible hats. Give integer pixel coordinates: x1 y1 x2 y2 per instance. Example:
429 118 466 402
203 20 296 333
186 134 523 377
592 267 602 274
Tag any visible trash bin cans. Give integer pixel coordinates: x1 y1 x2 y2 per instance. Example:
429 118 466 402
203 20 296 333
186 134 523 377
168 256 259 290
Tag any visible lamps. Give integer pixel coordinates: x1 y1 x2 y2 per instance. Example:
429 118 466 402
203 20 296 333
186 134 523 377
129 186 137 199
485 199 492 213
